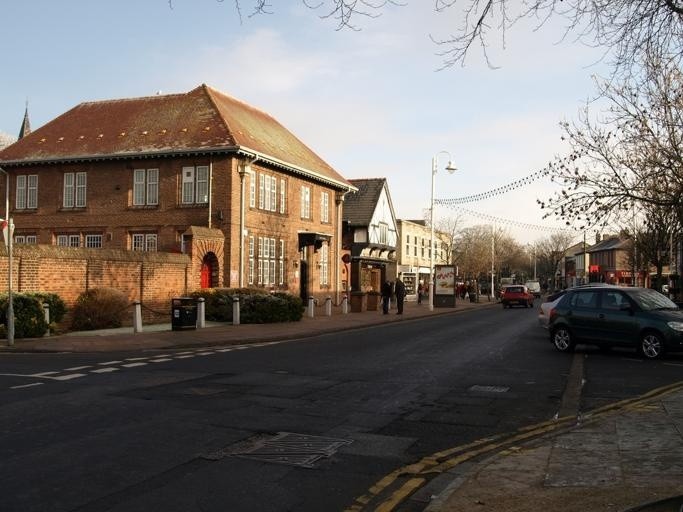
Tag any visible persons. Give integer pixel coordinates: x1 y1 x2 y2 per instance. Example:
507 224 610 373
416 284 422 304
455 280 473 300
380 279 391 314
394 278 405 315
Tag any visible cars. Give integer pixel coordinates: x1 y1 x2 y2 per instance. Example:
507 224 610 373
538 283 683 361
497 277 541 309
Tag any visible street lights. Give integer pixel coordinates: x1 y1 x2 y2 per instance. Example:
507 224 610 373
491 223 504 298
237 163 252 288
428 149 459 312
529 241 538 281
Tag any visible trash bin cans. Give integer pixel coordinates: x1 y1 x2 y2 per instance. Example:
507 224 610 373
172 297 197 330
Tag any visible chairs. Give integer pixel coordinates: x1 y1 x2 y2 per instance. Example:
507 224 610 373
608 295 629 309
574 297 585 304
